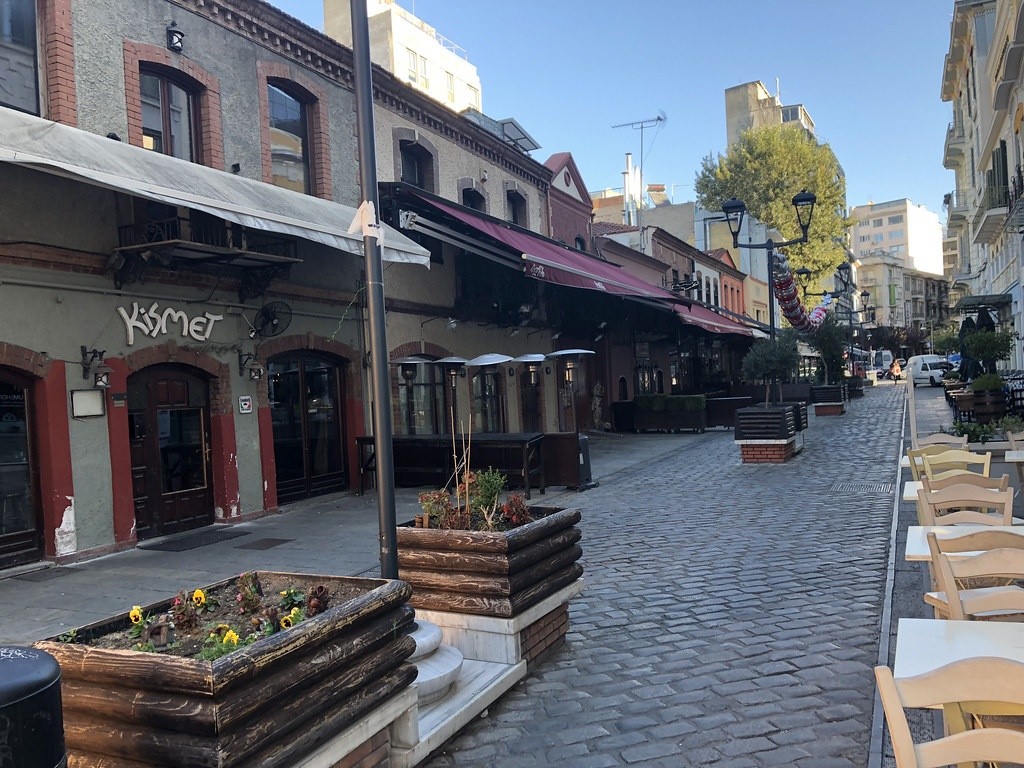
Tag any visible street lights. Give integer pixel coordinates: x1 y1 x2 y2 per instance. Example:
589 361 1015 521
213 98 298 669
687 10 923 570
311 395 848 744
797 261 852 386
834 290 870 388
722 188 816 409
848 306 875 385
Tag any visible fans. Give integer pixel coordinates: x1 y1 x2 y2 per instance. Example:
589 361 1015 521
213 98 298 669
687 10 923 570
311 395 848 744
249 301 292 340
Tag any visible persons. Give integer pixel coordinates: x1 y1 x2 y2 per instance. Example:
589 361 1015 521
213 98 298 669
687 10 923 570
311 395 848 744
889 358 901 385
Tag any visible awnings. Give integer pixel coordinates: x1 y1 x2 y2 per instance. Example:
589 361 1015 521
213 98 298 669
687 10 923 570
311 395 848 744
0 106 431 295
378 182 676 314
674 292 753 336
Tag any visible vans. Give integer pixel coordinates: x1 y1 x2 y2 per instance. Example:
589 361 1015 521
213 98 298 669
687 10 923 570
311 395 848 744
907 355 951 387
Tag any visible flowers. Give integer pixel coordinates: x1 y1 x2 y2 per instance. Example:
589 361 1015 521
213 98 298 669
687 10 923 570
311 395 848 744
127 604 151 639
184 587 221 615
278 607 308 629
278 588 305 610
194 620 256 661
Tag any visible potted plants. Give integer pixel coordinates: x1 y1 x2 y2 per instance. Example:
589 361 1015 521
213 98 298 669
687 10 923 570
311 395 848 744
756 333 808 431
806 313 845 405
813 318 849 401
734 338 796 440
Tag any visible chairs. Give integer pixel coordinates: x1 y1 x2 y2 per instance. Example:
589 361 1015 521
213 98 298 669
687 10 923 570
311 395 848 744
911 432 968 474
917 483 1024 590
921 471 1009 514
922 450 992 513
937 548 1024 737
1006 431 1024 495
906 444 970 525
874 654 1024 768
941 378 975 423
926 531 1024 619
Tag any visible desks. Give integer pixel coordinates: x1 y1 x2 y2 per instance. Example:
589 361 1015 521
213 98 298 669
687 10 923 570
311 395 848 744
900 452 979 468
356 433 545 500
1005 450 1024 463
947 382 972 390
905 525 1024 619
893 618 1024 768
903 480 999 504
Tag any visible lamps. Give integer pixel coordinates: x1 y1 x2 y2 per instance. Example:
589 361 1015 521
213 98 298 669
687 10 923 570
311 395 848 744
592 331 604 342
527 328 562 340
237 348 265 382
421 314 457 331
166 20 185 54
486 323 520 337
362 352 371 369
80 345 114 389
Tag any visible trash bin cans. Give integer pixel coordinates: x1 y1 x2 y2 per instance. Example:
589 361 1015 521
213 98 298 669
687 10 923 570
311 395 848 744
567 433 599 493
0 645 68 768
